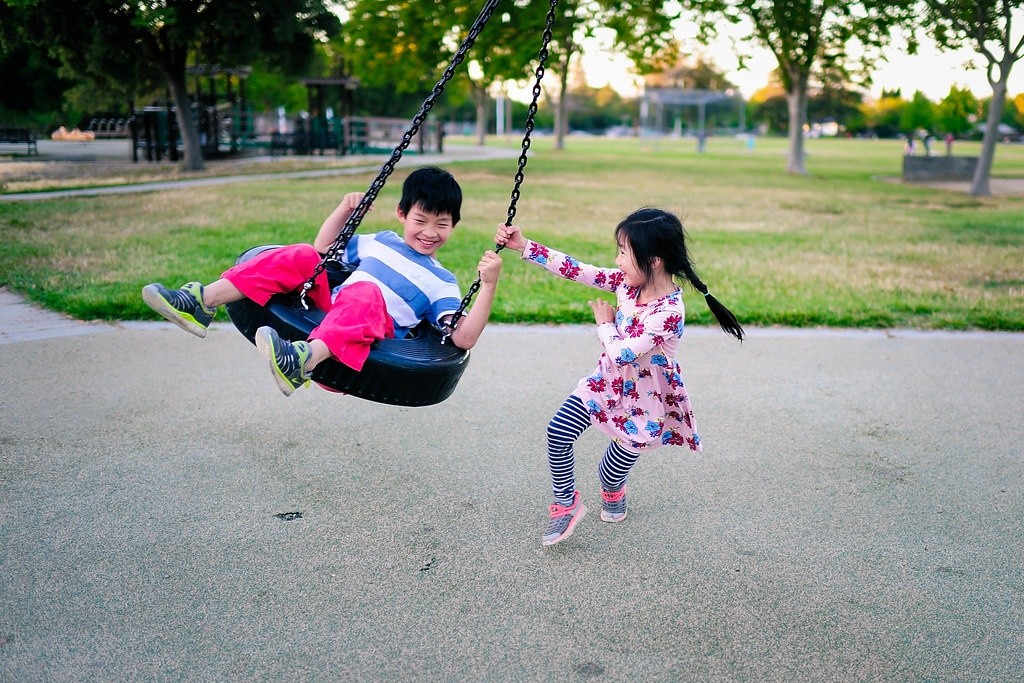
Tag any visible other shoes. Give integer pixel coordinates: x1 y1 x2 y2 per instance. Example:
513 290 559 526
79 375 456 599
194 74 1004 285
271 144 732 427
542 491 587 546
600 484 626 523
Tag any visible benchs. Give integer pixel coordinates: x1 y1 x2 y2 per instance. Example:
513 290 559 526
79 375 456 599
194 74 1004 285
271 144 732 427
0 129 39 155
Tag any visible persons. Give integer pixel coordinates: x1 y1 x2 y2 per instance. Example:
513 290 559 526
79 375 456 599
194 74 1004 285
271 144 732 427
903 125 954 157
493 206 702 546
142 166 503 397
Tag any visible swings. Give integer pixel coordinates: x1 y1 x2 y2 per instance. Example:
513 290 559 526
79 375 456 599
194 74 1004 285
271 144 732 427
225 0 557 409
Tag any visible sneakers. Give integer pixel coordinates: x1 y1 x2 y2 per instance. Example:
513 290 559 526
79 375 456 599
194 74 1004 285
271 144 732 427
143 282 216 337
256 327 313 396
